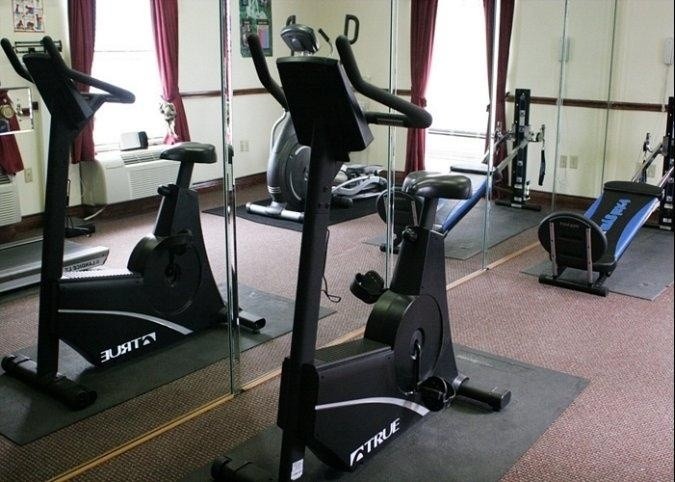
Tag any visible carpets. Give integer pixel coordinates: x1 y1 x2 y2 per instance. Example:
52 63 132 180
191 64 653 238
517 225 674 304
203 191 383 235
362 198 551 268
169 343 592 482
0 282 337 445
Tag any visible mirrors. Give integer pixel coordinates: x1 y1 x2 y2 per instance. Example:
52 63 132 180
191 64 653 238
388 1 484 287
551 2 616 221
482 1 570 269
227 0 390 395
0 0 236 482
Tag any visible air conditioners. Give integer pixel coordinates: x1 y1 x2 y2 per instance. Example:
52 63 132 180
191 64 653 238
80 141 194 207
0 169 23 227
